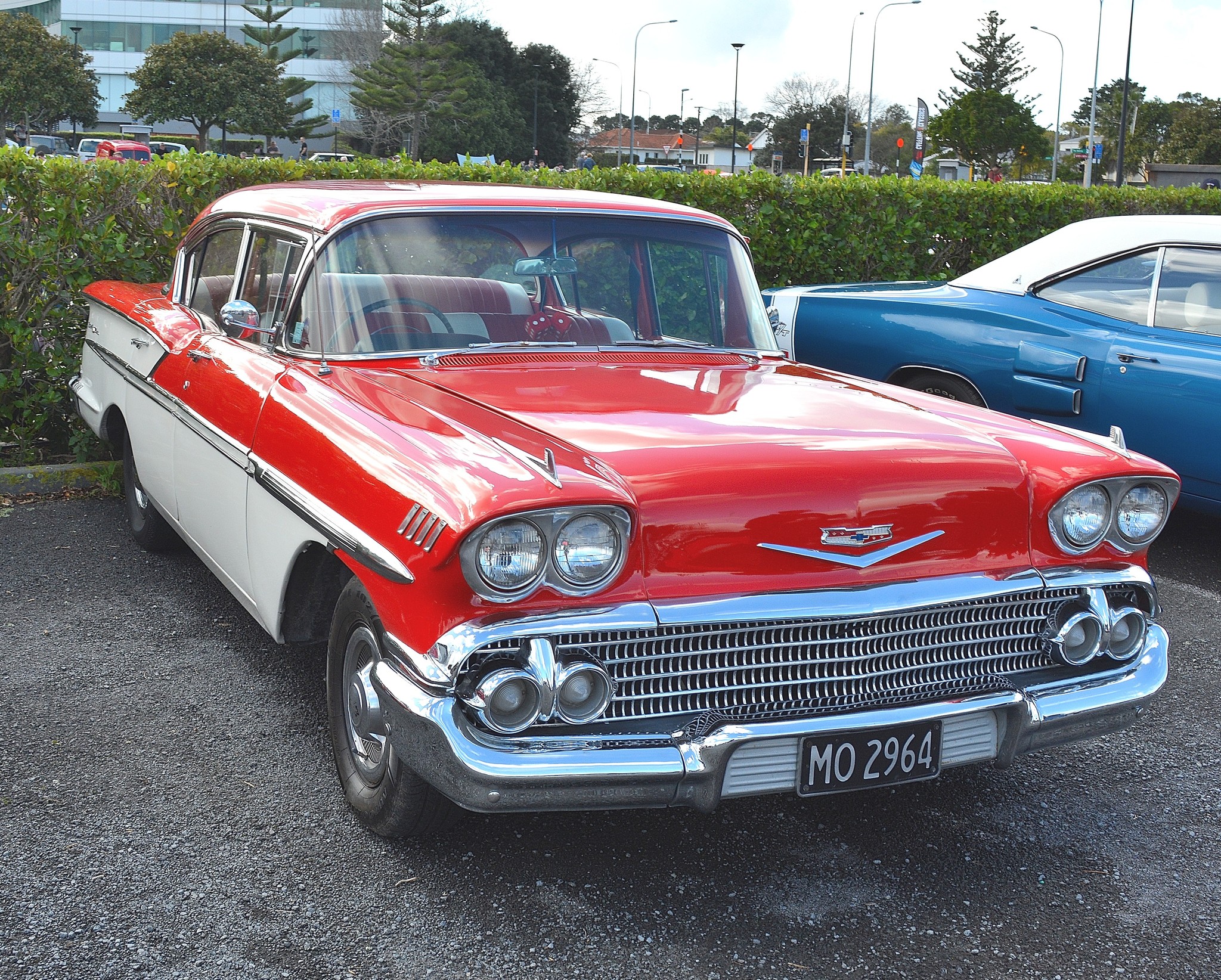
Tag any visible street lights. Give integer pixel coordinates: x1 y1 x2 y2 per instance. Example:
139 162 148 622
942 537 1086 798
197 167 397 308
592 58 623 167
629 20 679 164
863 0 922 179
679 88 689 169
1030 26 1064 183
639 90 651 118
730 43 746 173
841 12 864 177
69 26 84 151
531 64 543 164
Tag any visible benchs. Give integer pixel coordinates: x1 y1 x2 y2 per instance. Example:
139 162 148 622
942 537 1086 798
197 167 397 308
192 274 635 353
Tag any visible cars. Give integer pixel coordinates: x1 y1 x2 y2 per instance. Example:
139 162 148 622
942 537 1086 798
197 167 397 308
63 176 1192 846
759 212 1221 522
634 165 683 173
819 168 856 178
6 133 355 166
